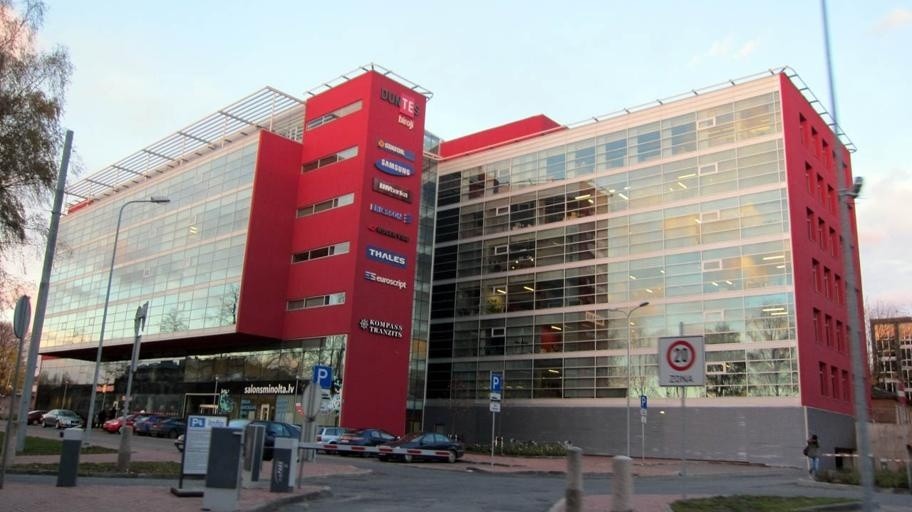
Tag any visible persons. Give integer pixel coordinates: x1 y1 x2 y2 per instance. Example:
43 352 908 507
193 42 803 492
804 434 821 479
98 405 107 427
109 403 116 422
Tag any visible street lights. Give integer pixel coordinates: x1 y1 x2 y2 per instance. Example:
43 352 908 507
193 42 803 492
606 300 652 456
83 196 171 441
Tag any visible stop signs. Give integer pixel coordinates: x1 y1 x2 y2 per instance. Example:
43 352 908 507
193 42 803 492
896 380 907 406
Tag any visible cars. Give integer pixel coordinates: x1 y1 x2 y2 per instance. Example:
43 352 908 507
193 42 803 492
334 426 399 458
104 410 186 439
313 424 351 454
375 430 463 465
172 419 302 461
29 408 48 425
40 407 84 429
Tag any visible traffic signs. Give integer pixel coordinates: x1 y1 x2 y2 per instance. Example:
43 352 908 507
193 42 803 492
657 334 705 387
489 392 502 401
489 402 501 412
